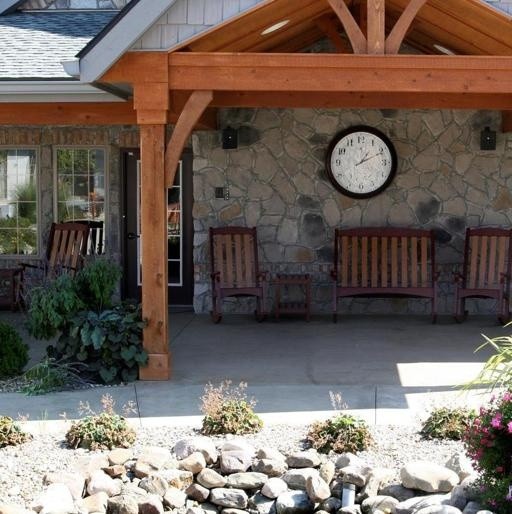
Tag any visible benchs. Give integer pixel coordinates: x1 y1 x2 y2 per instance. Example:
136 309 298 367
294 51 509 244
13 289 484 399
332 228 440 323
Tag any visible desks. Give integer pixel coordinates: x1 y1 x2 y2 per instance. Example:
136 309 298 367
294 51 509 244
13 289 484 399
0 265 25 306
270 272 312 320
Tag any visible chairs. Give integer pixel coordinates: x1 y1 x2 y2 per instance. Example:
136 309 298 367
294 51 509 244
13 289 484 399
61 219 103 257
456 225 511 327
21 219 104 307
206 224 266 322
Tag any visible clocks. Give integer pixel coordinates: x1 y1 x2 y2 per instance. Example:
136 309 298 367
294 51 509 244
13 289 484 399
323 124 398 200
